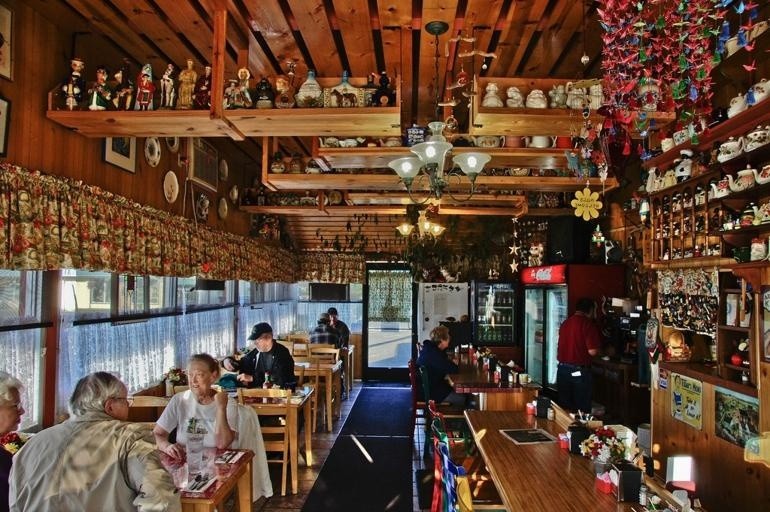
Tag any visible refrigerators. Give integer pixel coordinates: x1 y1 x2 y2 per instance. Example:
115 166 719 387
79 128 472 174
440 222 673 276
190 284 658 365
522 262 628 404
469 280 518 345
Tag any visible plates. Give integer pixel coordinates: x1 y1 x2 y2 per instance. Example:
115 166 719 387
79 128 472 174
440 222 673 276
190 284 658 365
165 137 180 153
218 160 230 181
145 137 160 167
218 198 228 219
163 171 180 203
229 184 239 207
292 392 306 398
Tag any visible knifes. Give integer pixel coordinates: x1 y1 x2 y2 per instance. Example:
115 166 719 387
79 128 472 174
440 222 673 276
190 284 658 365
225 451 238 463
197 473 217 489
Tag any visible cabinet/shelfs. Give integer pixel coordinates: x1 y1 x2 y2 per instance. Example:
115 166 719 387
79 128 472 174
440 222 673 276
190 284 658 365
641 23 770 511
44 7 621 219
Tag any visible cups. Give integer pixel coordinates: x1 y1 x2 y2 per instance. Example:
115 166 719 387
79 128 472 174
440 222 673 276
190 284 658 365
368 143 376 146
214 462 231 478
186 435 203 472
165 462 188 489
519 373 528 385
285 382 296 393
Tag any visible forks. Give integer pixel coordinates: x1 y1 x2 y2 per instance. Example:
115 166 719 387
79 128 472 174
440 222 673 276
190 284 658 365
192 473 209 490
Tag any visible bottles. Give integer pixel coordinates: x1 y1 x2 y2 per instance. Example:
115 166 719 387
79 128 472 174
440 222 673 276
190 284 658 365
374 72 395 106
299 71 322 102
639 486 647 505
478 293 512 306
547 407 554 420
477 326 512 343
508 373 514 382
365 74 376 90
263 371 272 404
477 309 512 325
257 187 265 205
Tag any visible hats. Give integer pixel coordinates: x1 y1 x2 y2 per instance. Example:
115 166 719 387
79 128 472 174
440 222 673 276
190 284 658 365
320 313 330 320
247 322 272 340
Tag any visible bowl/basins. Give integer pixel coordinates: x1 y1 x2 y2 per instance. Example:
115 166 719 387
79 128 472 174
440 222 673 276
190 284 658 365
490 168 509 176
509 168 531 177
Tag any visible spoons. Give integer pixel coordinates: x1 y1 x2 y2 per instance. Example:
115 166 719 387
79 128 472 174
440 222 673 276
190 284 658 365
188 474 202 488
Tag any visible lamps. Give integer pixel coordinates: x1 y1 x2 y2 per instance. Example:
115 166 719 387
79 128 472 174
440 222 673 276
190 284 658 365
396 208 448 249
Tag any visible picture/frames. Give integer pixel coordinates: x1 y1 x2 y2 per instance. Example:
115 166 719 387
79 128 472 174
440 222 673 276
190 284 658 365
0 94 13 158
1 0 16 84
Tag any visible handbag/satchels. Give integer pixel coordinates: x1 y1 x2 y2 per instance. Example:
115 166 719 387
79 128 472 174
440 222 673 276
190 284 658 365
218 373 245 390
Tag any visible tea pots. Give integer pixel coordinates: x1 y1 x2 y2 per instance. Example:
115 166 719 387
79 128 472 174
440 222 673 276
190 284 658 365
469 135 572 148
339 138 366 148
711 75 770 263
379 136 403 147
317 136 339 148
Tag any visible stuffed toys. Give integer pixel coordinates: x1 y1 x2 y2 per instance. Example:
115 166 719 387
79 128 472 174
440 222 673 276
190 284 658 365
528 242 545 268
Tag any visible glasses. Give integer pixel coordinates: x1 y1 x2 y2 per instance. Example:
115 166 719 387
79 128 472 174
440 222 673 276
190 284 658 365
111 395 134 408
2 401 24 412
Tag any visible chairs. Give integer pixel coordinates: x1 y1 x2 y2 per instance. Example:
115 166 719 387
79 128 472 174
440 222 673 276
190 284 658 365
408 341 701 512
9 327 356 512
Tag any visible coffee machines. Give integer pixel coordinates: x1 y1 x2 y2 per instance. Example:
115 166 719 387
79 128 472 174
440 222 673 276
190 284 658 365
598 297 641 362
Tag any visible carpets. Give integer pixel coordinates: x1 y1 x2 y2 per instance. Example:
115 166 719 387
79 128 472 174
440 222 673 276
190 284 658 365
302 385 416 512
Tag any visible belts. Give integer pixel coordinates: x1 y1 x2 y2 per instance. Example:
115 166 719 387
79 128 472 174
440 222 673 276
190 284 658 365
559 363 582 369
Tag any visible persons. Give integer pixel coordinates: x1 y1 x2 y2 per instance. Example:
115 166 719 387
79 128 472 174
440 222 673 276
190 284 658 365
557 299 603 413
0 371 181 512
414 325 475 438
59 56 297 110
153 354 239 457
217 322 295 467
311 308 349 397
